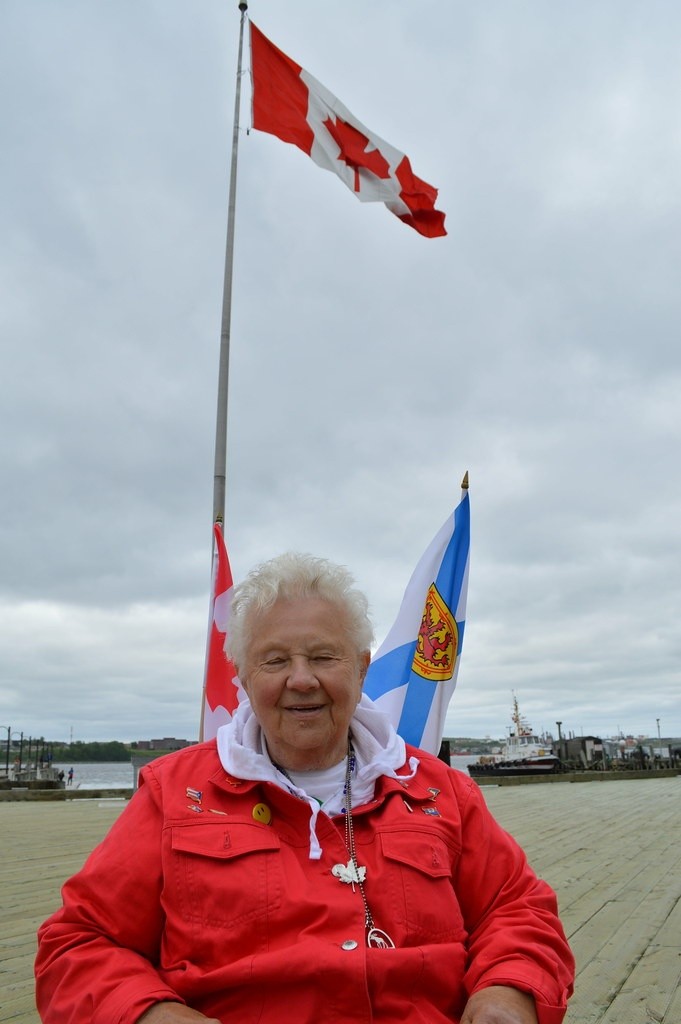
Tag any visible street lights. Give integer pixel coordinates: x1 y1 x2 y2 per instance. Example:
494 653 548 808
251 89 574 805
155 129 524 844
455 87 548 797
9 731 32 772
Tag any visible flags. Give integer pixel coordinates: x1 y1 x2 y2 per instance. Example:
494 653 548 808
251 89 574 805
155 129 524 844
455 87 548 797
246 15 447 238
199 511 249 748
360 470 473 758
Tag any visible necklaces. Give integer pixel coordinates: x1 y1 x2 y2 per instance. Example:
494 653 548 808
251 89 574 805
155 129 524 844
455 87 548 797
265 731 397 949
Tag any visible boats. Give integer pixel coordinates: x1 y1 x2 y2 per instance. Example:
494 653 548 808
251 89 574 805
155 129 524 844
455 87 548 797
465 687 560 778
15 767 66 789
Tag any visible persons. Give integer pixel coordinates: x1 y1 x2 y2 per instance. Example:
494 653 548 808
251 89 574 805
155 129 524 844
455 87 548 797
40 754 53 768
14 754 20 772
34 547 577 1024
67 767 73 786
58 770 64 781
